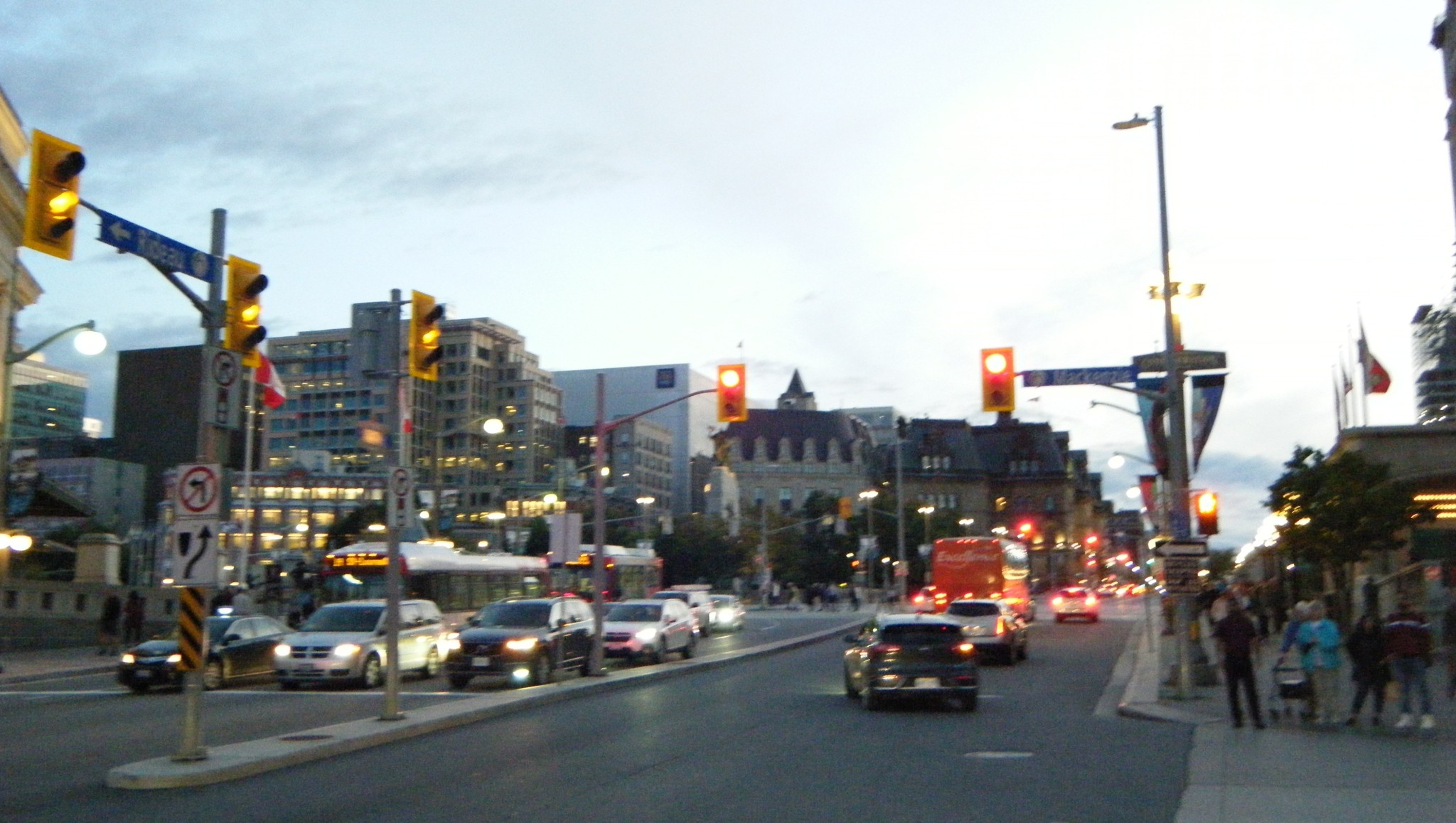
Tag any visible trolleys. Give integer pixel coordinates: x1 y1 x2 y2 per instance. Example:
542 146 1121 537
1265 655 1315 726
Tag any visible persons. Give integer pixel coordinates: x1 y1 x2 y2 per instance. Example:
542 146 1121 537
1187 567 1436 730
94 583 121 656
123 588 143 648
732 568 899 612
210 561 317 628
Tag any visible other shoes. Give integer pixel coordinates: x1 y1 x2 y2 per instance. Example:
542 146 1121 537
1392 714 1414 728
1418 714 1437 730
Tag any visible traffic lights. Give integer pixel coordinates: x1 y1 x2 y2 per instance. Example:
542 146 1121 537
408 292 445 381
717 365 747 423
840 497 853 518
1192 492 1218 535
19 128 85 260
982 347 1015 411
223 254 268 354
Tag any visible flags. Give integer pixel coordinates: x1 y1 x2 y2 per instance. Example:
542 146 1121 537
1357 339 1390 394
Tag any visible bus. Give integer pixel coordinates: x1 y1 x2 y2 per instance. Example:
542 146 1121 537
320 542 549 630
932 537 1035 615
545 555 666 602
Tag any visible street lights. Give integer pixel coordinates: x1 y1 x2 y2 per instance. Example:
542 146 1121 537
516 492 557 555
860 490 878 604
434 412 503 537
0 321 106 581
918 506 935 584
958 519 974 537
1113 107 1198 699
635 497 655 547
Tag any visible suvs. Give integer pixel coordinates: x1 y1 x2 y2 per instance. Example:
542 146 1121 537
939 598 1030 662
442 597 597 689
273 598 451 690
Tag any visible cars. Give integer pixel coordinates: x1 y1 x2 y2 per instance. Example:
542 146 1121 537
1050 587 1103 623
841 613 981 710
599 585 747 666
914 587 937 609
1093 582 1147 597
117 616 296 693
